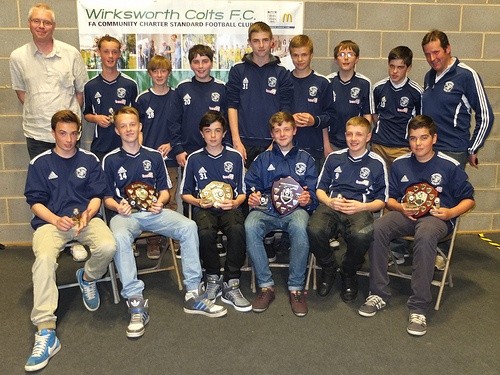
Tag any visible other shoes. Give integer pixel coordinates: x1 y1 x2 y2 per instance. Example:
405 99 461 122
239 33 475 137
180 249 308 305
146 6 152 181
342 288 354 303
316 280 331 299
328 237 340 247
434 254 446 270
388 255 405 266
175 249 181 259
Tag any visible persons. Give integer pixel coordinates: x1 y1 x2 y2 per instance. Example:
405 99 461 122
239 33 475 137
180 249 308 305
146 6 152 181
244 112 318 317
228 21 294 262
421 28 494 271
134 55 175 259
8 3 90 261
83 36 140 257
102 107 228 339
358 115 475 335
306 117 389 304
286 35 340 248
180 111 252 312
372 46 425 266
325 40 375 154
25 109 118 371
172 44 227 259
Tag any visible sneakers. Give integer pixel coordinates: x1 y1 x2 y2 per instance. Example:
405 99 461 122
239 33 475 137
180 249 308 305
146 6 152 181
125 297 150 338
358 294 386 316
183 281 228 318
265 243 277 262
216 241 226 257
221 278 252 311
204 273 223 304
131 242 140 256
289 290 308 317
406 312 427 335
146 239 162 259
75 268 100 312
24 328 61 371
252 287 275 312
70 245 89 262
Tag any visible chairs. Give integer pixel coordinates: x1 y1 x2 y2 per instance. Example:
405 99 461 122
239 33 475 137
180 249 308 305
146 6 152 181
58 165 459 311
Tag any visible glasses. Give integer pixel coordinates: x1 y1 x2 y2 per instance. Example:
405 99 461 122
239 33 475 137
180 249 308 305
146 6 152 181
337 52 357 58
